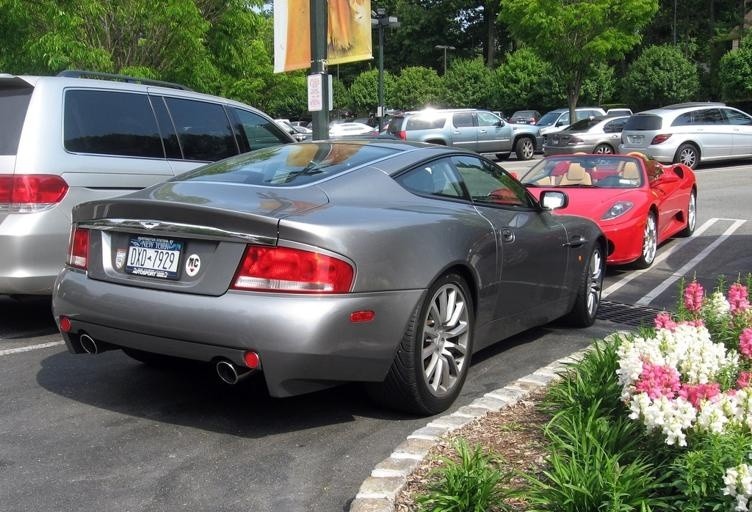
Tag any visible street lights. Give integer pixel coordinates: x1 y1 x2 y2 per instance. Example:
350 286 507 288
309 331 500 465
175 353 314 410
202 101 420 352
368 6 397 132
435 45 456 76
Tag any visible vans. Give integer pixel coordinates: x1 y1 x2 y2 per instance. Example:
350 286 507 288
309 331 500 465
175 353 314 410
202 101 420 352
0 68 303 305
535 107 607 144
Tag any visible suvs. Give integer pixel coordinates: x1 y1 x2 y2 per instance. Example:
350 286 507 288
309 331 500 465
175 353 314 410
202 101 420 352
387 108 542 161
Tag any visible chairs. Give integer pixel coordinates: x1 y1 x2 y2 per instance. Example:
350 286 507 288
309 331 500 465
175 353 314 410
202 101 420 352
557 161 592 186
615 161 642 187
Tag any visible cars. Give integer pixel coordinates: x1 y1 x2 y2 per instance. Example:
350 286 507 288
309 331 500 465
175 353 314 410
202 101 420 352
542 116 627 161
608 108 633 119
270 106 408 142
509 110 540 126
490 111 508 126
618 102 752 169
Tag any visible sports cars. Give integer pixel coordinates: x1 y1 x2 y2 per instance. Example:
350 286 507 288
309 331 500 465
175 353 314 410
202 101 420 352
56 139 607 414
488 152 698 271
